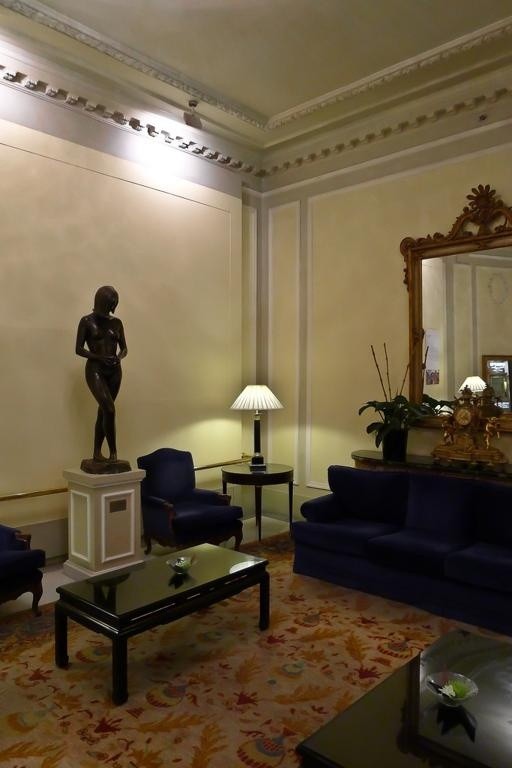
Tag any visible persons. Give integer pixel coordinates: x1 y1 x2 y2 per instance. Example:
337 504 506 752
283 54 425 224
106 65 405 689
75 286 128 462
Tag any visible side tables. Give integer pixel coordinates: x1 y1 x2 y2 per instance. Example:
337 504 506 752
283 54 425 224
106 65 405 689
221 463 294 544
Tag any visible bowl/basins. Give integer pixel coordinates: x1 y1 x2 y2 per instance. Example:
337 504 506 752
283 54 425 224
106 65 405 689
166 556 197 575
425 672 478 706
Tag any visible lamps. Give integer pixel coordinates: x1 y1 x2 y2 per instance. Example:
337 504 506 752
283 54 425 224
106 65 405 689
229 384 285 471
458 376 488 398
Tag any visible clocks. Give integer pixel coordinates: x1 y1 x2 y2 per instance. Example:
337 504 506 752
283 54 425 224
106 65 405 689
430 386 509 465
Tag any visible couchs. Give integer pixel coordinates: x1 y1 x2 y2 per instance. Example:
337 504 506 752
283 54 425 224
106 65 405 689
291 464 512 638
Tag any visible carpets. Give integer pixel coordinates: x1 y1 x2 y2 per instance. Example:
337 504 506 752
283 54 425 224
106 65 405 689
0 529 512 768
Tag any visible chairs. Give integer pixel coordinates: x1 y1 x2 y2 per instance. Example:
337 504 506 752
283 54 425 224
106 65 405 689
137 448 244 555
0 523 44 617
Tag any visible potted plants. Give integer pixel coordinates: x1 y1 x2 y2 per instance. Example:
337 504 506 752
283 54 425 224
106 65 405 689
358 342 423 463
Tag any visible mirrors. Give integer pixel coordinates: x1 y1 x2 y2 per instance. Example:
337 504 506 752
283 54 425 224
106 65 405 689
400 184 512 435
482 354 512 415
